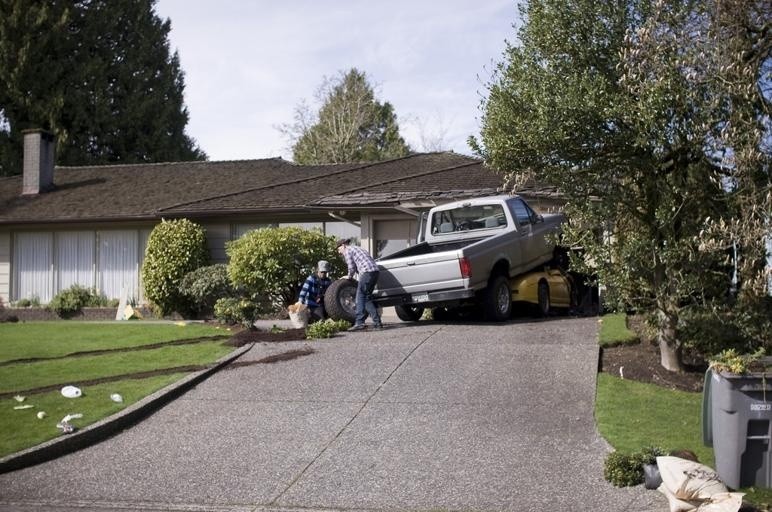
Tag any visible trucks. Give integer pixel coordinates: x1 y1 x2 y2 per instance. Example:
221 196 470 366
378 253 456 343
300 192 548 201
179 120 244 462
363 191 572 311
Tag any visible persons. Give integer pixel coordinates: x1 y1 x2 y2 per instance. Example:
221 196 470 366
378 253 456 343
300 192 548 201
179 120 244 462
335 239 382 332
295 260 332 320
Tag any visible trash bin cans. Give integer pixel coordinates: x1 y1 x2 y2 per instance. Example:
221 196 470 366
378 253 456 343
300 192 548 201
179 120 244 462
702 356 772 491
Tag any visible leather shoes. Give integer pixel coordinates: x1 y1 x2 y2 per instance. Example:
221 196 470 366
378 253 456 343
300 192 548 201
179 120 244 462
347 323 384 331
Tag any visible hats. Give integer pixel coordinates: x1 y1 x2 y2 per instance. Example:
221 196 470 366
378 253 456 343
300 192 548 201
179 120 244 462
334 238 351 250
317 260 329 272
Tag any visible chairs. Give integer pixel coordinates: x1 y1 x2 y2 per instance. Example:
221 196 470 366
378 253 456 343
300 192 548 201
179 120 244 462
485 216 500 228
440 223 454 232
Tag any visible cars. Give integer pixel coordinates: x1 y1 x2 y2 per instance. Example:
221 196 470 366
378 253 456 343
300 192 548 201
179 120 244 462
432 259 602 321
